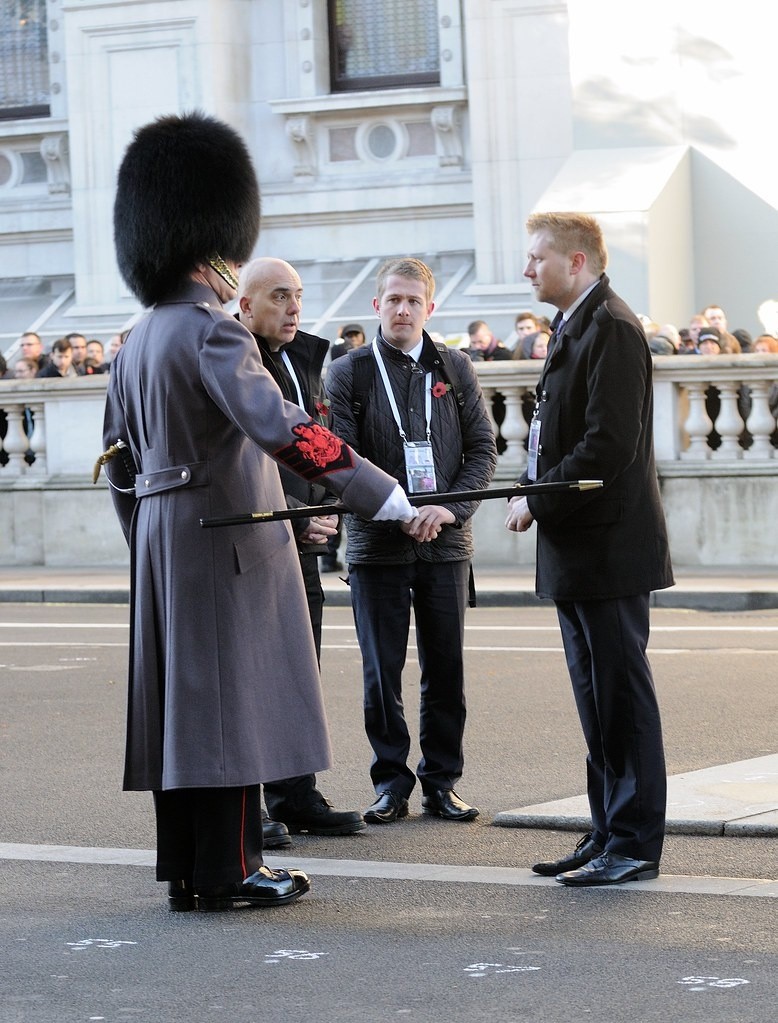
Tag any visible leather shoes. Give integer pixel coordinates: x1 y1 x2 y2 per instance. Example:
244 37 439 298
531 831 603 877
286 798 368 837
363 788 409 823
194 865 310 911
421 790 480 821
167 879 199 911
554 850 660 887
260 808 292 847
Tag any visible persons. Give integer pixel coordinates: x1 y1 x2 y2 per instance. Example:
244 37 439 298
231 257 368 846
0 304 778 573
323 257 498 824
505 212 676 887
101 106 421 912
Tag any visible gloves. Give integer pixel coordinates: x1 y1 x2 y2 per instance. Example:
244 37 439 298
372 482 420 523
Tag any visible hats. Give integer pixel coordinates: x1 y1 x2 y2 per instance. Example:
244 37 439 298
698 326 723 349
341 324 363 337
112 108 261 309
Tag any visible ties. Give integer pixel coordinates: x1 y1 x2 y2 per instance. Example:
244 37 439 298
556 318 567 341
